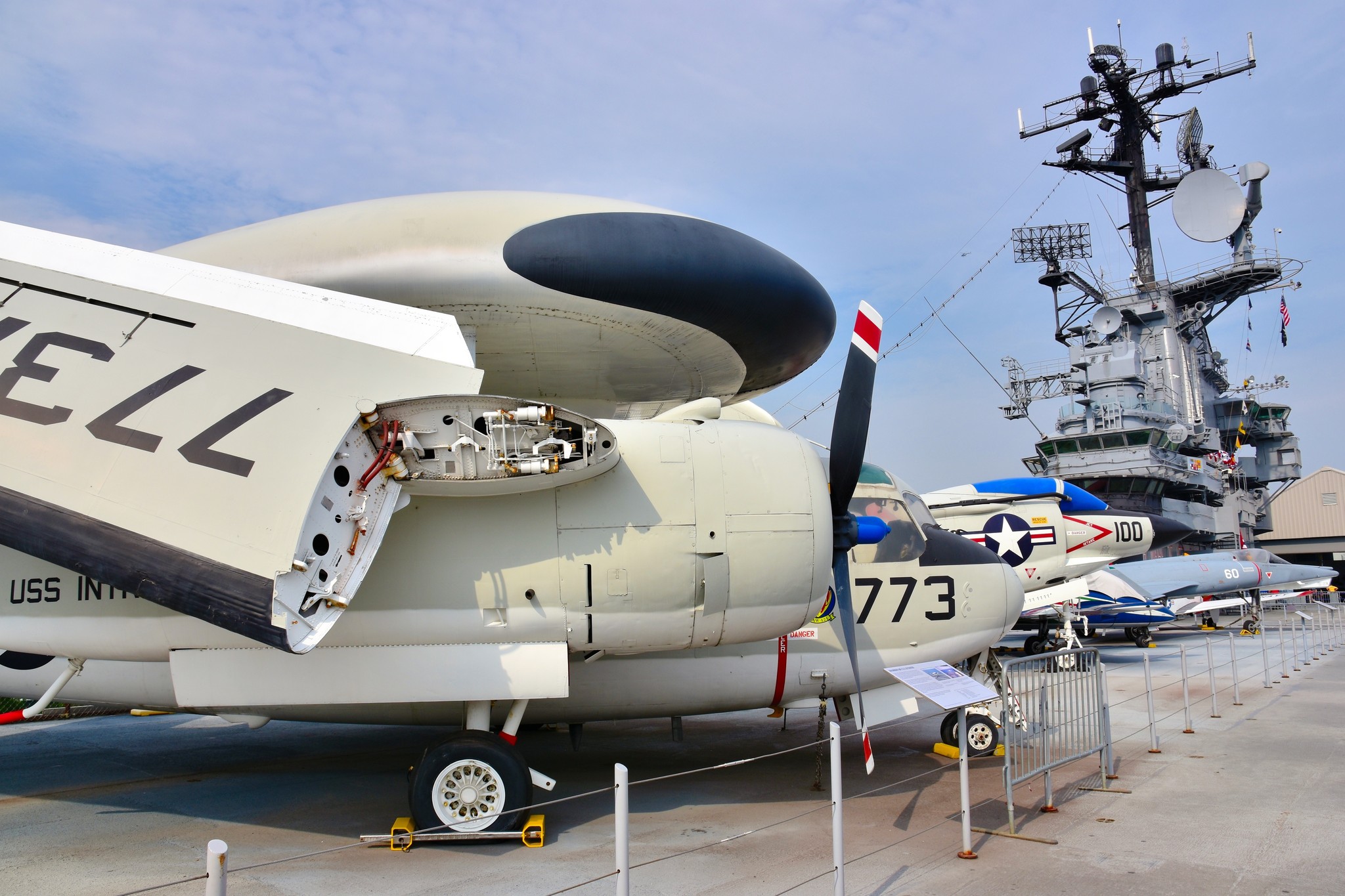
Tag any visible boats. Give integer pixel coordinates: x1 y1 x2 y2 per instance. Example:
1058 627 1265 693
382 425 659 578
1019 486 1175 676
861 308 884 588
996 17 1311 564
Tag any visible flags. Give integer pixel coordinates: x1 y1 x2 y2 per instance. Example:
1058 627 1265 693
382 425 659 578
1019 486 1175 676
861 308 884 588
1279 317 1287 348
1244 379 1249 390
1242 400 1248 415
1205 450 1235 471
1246 338 1252 352
1239 531 1248 550
1235 436 1241 449
1248 296 1252 310
1248 317 1252 331
1280 289 1290 326
1238 420 1246 435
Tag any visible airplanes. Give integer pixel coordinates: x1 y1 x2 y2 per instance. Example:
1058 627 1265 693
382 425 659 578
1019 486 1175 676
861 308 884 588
0 185 1345 848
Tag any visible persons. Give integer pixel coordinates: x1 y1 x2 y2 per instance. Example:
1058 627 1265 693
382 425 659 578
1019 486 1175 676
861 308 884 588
1315 588 1324 603
1309 589 1315 600
1327 584 1338 592
